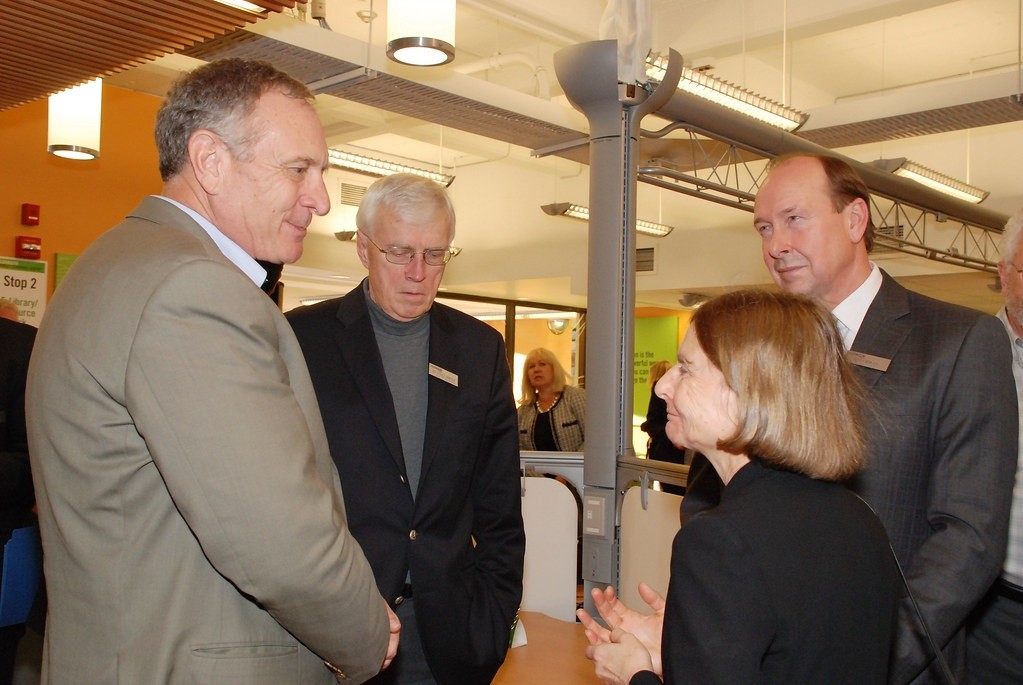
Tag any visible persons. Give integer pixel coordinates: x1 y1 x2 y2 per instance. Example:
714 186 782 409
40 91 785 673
516 346 586 489
23 58 402 685
280 175 527 685
754 154 1023 685
962 211 1023 685
640 361 686 497
575 291 903 684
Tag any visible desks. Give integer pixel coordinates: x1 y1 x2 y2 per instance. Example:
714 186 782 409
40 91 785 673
491 611 612 685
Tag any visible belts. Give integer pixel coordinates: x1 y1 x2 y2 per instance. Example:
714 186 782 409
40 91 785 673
401 582 413 599
991 578 1023 604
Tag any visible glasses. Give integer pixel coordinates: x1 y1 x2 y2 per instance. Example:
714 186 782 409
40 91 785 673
362 232 454 266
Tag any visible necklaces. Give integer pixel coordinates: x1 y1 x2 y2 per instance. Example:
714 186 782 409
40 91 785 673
536 395 558 413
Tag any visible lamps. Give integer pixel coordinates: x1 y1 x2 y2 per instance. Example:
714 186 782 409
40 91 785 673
869 18 991 205
385 0 456 67
47 76 103 160
541 156 674 238
678 293 711 309
327 125 456 188
